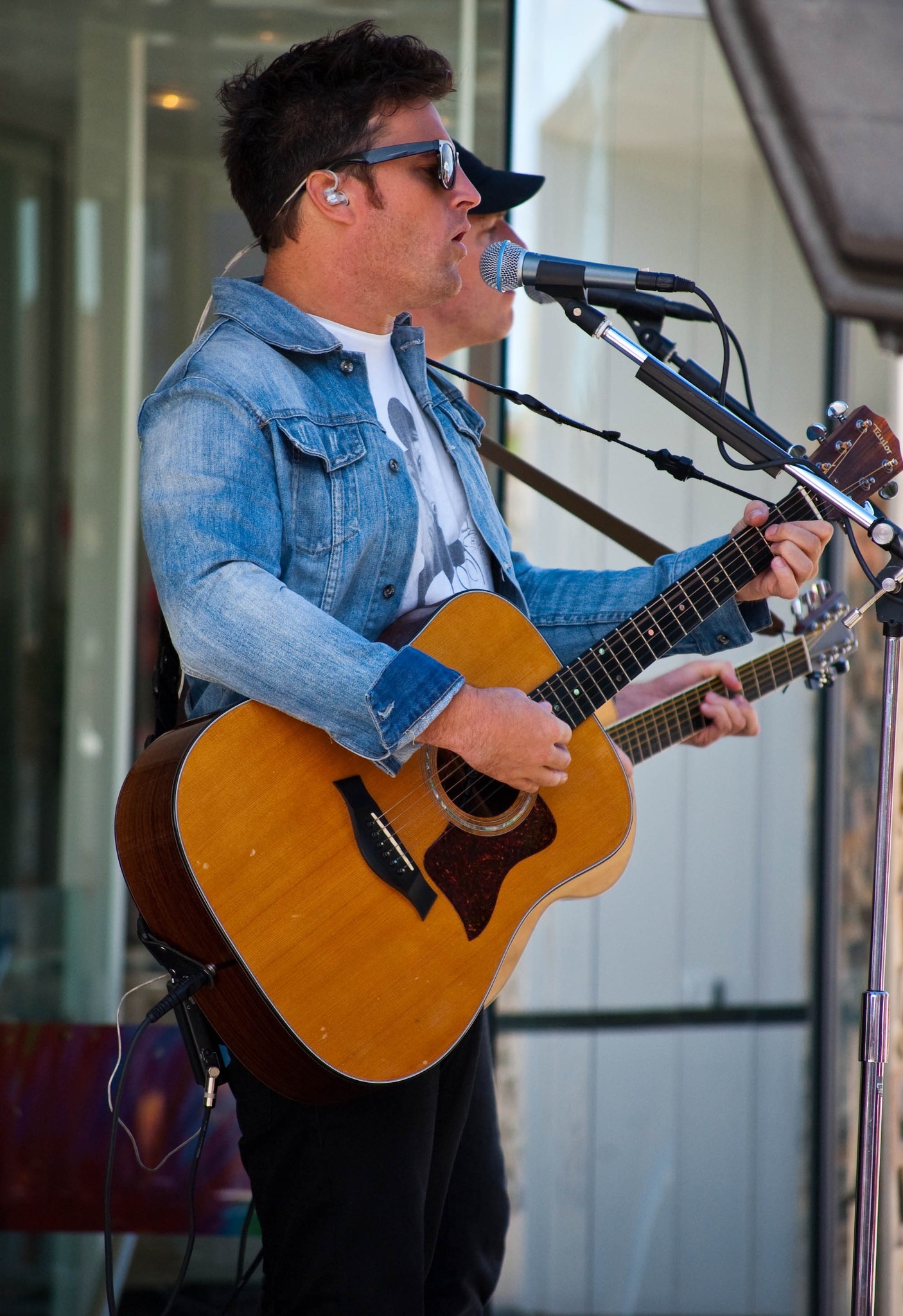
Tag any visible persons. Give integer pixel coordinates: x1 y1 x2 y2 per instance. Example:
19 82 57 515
135 18 831 1316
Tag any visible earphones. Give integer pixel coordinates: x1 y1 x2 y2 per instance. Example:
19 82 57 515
324 189 351 206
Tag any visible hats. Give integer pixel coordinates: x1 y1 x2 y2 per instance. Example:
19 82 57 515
452 135 547 216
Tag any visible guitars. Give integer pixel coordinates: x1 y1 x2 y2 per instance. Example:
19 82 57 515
109 396 903 1090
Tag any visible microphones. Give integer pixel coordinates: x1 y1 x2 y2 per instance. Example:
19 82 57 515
523 285 713 324
479 240 694 293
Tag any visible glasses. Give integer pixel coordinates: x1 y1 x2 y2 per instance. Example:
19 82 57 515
328 138 461 190
